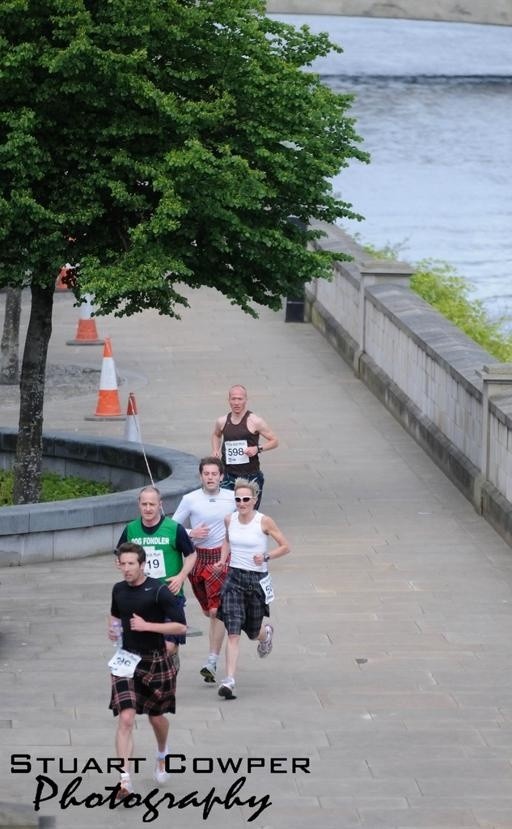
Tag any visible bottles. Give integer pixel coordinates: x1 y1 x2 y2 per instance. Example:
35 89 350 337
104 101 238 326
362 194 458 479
112 622 123 648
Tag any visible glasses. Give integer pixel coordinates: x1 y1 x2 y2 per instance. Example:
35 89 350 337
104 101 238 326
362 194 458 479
235 496 256 503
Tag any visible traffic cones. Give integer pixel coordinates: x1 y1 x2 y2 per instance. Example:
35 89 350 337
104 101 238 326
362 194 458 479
63 284 106 346
84 338 129 422
124 392 141 442
56 266 68 288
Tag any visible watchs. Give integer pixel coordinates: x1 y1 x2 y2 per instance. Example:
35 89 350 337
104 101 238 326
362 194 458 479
262 552 271 562
256 444 263 454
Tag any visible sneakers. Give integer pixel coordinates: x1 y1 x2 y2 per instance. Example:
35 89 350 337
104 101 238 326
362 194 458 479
116 781 134 804
256 622 273 659
200 663 217 683
218 683 237 699
154 745 170 786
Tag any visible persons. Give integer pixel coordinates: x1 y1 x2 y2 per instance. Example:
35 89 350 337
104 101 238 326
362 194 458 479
111 486 198 679
103 541 189 803
209 383 278 512
170 454 241 683
211 476 292 701
